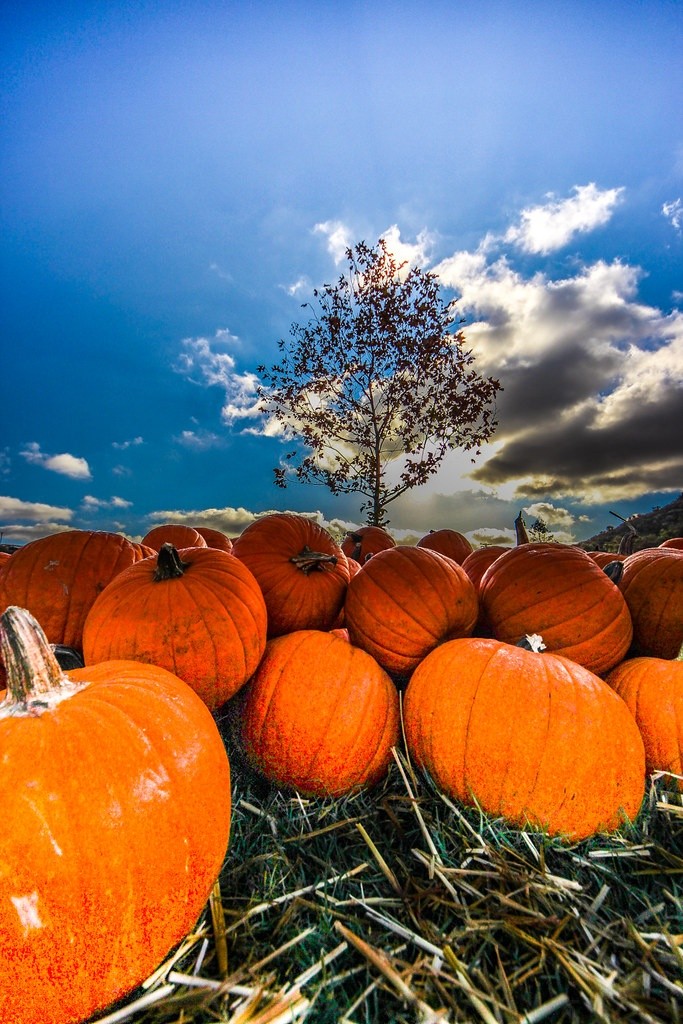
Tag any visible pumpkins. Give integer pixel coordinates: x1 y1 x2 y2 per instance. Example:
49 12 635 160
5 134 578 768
0 606 232 1024
0 512 683 842
